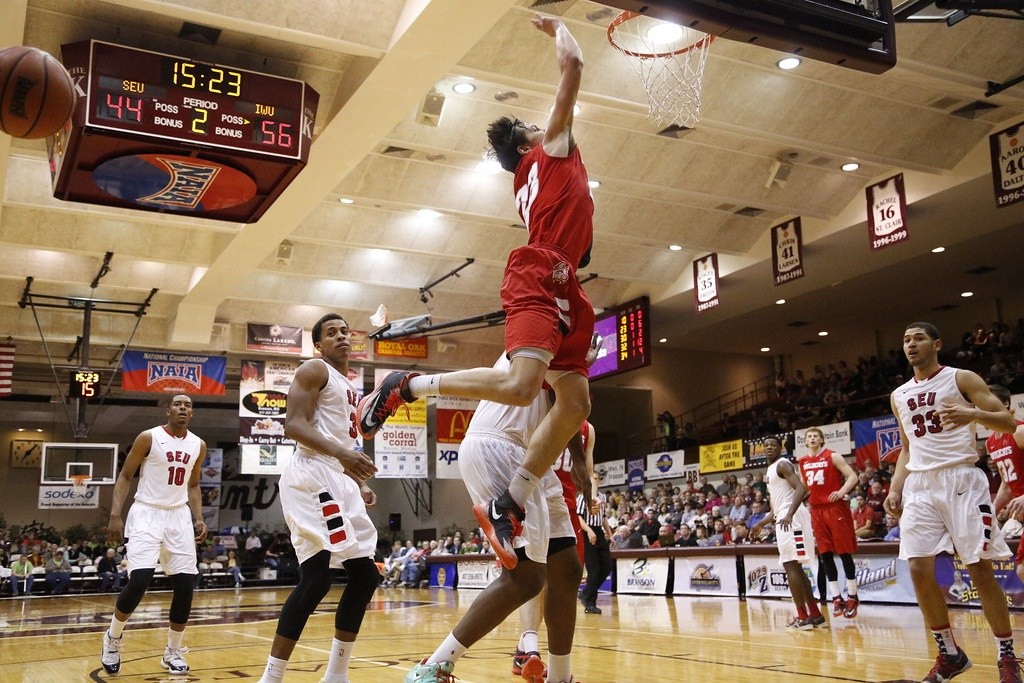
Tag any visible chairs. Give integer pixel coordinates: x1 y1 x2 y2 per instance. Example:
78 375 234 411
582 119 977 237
0 545 237 597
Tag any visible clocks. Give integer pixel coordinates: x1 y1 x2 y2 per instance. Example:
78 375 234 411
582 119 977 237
10 438 45 469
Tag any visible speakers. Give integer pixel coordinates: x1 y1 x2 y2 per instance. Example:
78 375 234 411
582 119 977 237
389 513 401 531
765 159 794 189
240 503 253 521
414 92 446 128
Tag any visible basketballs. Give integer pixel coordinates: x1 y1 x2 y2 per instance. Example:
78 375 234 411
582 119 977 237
0 44 79 142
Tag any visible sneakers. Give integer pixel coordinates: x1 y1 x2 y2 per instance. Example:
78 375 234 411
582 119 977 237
101 629 122 676
922 646 972 683
160 644 190 675
997 653 1024 683
356 370 421 440
472 489 526 570
404 657 460 683
832 593 860 618
512 646 548 683
786 614 828 630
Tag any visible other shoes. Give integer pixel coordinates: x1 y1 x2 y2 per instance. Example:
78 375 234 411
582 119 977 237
578 593 591 611
584 605 601 614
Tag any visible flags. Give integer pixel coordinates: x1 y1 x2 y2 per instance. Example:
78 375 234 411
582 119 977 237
0 343 16 395
121 348 227 395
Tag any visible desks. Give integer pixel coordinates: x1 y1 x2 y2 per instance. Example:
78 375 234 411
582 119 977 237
426 536 1024 616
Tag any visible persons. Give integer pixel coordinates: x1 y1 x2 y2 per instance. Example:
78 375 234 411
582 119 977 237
0 523 299 597
258 314 379 683
101 392 209 677
405 330 612 683
356 11 594 570
752 435 828 630
986 384 1024 585
380 318 1024 588
883 322 1024 683
798 428 860 619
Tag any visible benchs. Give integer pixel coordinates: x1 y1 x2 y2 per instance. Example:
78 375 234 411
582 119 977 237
206 531 391 589
